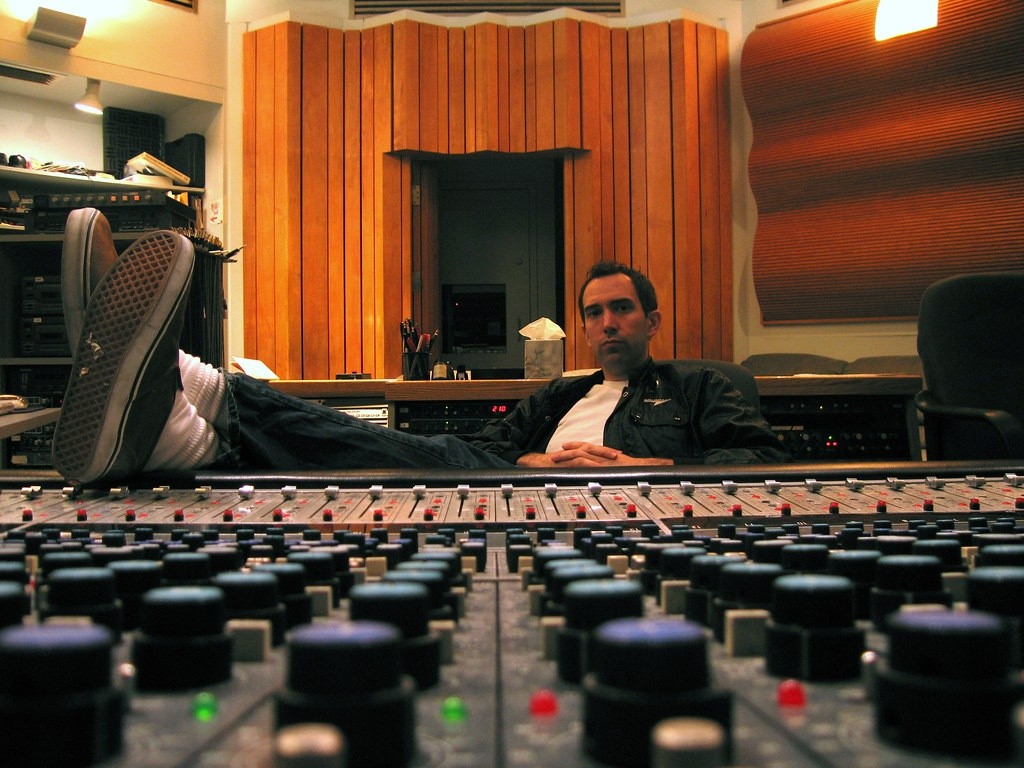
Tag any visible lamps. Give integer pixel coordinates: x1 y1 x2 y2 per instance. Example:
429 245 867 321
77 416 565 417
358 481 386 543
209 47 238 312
24 6 86 50
74 78 105 115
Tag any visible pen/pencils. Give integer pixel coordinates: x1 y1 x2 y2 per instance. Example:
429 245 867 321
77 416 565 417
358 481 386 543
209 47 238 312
399 317 440 379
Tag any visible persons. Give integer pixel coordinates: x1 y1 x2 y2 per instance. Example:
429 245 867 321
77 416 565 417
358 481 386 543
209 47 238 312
51 206 782 483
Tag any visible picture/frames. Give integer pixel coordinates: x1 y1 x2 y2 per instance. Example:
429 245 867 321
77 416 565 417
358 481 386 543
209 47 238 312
147 0 198 15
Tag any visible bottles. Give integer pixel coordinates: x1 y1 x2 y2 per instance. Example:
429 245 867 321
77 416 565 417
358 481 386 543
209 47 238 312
432 361 450 380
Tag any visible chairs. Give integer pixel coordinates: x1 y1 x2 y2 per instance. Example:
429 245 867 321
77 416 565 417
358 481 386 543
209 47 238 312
915 272 1024 460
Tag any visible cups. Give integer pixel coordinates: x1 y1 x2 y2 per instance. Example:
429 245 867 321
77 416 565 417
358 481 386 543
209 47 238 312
400 351 431 381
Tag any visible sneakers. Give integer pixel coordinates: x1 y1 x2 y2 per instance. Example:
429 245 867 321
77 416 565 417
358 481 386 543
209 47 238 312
51 229 196 490
61 207 119 363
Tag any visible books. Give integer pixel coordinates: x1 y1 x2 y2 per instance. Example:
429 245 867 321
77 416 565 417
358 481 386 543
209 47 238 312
121 153 191 186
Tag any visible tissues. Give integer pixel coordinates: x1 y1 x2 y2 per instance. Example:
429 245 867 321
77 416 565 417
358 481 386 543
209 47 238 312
518 316 567 380
